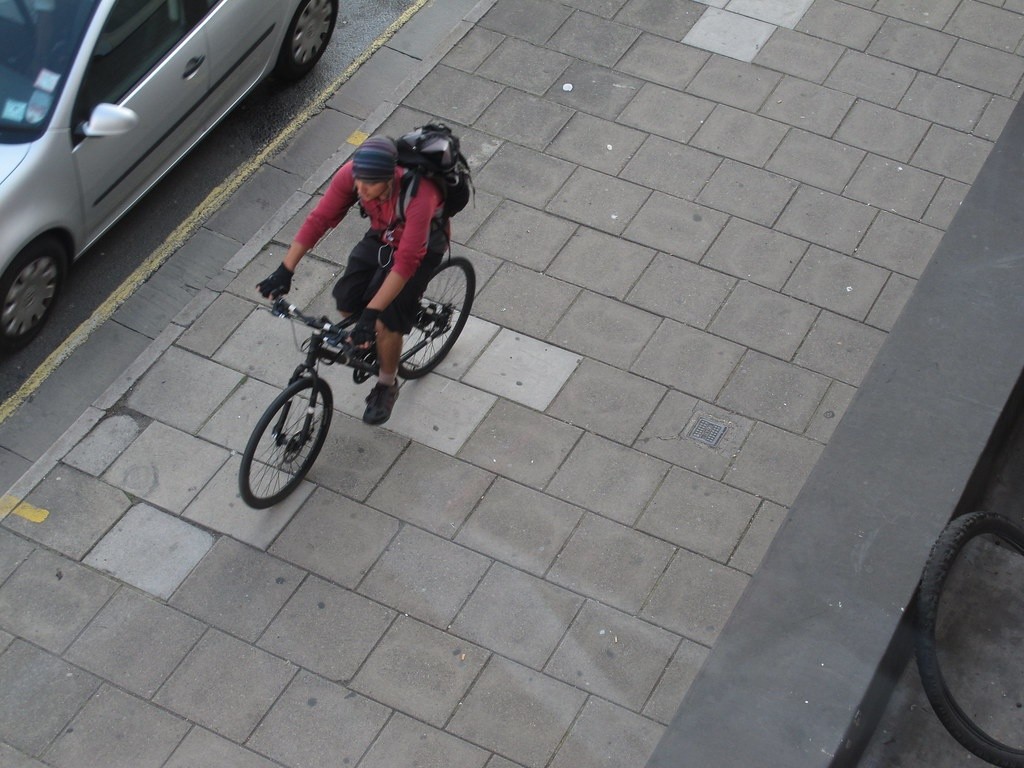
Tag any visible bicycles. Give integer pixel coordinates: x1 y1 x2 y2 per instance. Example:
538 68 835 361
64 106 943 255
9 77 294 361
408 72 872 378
236 253 481 511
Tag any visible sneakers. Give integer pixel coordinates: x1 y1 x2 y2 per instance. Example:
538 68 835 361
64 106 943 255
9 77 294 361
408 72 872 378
363 377 399 425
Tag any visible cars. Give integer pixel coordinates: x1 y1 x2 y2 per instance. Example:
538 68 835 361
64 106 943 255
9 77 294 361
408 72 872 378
0 0 343 351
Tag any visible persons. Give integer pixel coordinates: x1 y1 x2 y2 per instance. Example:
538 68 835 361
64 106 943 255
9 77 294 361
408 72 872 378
255 134 454 427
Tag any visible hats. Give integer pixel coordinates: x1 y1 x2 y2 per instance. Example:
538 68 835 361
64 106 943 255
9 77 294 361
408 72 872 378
353 137 398 182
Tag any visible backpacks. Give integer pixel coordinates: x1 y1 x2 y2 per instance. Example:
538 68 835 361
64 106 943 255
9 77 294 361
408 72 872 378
397 124 470 223
